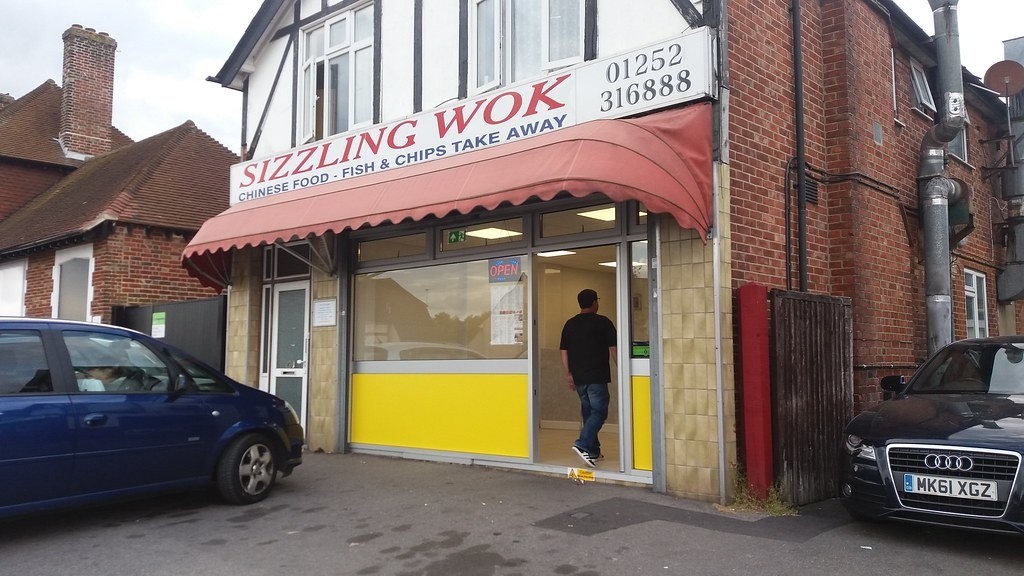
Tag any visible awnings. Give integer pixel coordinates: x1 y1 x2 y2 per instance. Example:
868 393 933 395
179 102 713 295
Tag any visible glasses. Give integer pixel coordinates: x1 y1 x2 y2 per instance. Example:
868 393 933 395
596 297 600 301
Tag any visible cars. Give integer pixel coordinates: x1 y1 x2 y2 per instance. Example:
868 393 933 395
0 316 304 522
366 343 486 360
838 336 1024 536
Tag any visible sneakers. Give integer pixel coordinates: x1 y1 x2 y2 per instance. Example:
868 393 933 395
594 454 605 461
571 446 596 467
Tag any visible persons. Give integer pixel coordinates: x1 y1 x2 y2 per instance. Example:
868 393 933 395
74 356 123 391
559 289 617 468
